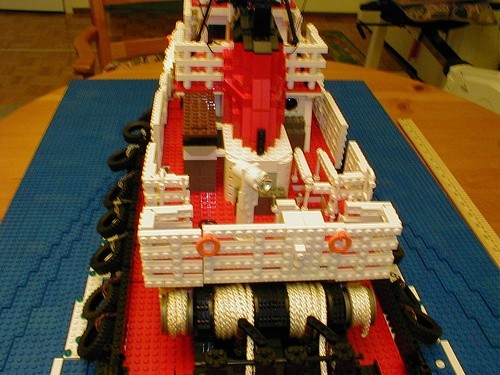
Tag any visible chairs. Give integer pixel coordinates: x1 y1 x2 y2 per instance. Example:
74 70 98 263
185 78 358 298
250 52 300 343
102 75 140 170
73 0 185 82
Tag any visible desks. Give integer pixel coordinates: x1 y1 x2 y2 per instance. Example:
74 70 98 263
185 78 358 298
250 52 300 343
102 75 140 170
0 54 500 375
